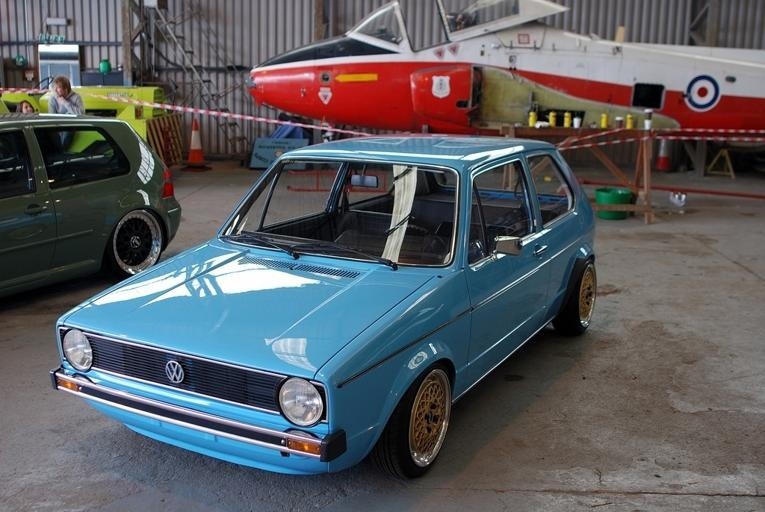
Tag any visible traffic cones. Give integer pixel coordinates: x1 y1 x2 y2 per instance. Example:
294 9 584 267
656 140 672 171
178 119 214 173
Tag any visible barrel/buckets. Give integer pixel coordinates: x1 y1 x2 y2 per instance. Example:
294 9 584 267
595 188 631 219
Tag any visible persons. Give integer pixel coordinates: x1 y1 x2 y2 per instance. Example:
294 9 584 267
48 76 85 152
16 100 34 114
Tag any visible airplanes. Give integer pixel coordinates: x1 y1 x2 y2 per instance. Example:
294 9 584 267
249 1 765 198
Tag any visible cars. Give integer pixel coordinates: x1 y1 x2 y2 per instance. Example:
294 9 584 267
1 119 185 302
50 136 602 478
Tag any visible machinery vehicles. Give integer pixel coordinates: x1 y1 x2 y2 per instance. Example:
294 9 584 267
0 74 165 160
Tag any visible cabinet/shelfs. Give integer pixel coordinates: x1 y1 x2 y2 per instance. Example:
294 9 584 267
81 68 122 86
38 43 82 88
